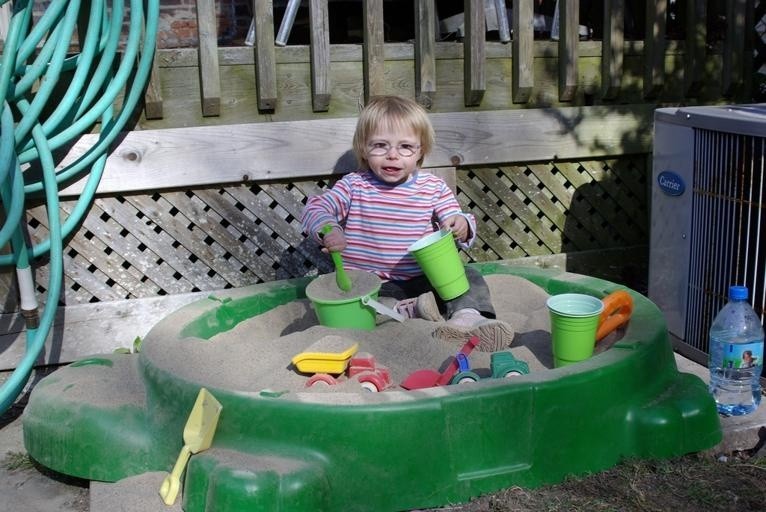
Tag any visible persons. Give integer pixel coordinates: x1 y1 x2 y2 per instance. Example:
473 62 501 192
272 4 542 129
297 93 478 282
738 349 758 370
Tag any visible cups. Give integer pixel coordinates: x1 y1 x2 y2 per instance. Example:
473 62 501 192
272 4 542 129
544 291 605 369
405 225 470 303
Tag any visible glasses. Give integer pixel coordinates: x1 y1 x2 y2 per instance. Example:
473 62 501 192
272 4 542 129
366 139 423 157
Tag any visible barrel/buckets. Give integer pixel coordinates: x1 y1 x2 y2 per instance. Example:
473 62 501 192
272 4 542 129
305 269 406 329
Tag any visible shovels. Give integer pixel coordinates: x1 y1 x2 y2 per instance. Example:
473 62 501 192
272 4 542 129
157 386 224 506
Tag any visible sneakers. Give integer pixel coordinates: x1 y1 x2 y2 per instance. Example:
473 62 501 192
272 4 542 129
392 291 442 321
432 313 515 352
